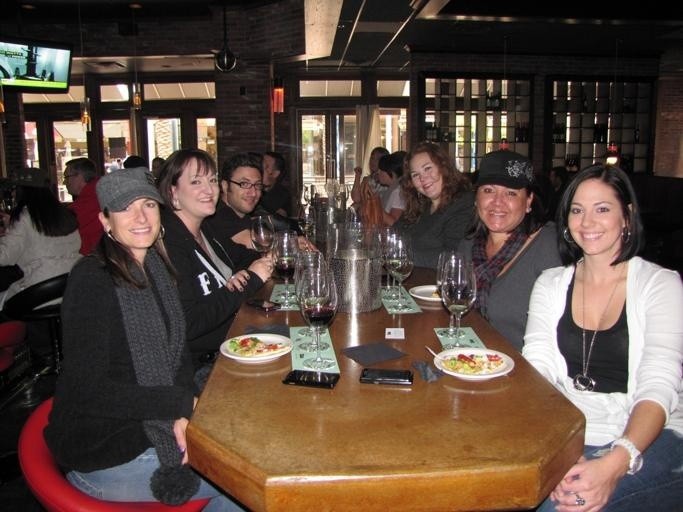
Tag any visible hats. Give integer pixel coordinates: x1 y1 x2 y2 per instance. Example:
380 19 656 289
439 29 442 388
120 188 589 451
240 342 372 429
15 168 50 188
477 149 536 190
96 167 165 211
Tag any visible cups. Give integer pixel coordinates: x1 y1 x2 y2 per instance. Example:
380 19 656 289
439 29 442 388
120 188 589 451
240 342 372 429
386 239 414 315
436 251 469 340
380 226 404 303
300 272 338 369
294 250 325 339
248 214 276 258
441 258 476 350
276 232 298 309
296 258 331 353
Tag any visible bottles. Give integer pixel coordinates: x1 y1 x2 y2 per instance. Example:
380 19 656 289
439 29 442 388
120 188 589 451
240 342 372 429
486 89 500 106
621 150 634 172
634 121 640 145
550 120 567 145
564 150 579 172
583 94 588 113
425 121 439 141
595 122 607 145
603 142 621 168
516 119 528 142
498 138 510 153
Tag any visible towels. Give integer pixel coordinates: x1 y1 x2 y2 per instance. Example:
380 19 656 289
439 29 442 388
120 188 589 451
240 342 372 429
245 322 289 338
381 274 399 286
410 360 442 382
274 269 295 283
342 342 408 365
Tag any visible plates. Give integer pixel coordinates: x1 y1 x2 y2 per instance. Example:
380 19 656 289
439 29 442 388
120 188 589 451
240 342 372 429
219 332 293 365
408 282 441 302
433 346 516 384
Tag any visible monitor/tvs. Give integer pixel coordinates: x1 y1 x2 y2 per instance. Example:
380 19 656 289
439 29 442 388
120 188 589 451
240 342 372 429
1 41 74 94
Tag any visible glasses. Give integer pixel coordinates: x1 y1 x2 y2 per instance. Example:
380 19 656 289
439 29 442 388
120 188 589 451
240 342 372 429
64 175 74 178
225 178 264 189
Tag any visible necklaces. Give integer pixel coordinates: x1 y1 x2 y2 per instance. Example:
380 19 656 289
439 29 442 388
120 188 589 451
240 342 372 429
574 255 628 391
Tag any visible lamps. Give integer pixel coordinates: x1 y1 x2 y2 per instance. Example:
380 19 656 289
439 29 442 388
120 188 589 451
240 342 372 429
81 69 93 132
131 62 144 110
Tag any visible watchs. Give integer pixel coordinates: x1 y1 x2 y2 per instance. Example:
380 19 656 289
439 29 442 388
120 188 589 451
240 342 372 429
612 439 642 475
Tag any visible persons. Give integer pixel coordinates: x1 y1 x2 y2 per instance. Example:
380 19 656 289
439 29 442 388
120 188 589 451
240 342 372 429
258 153 291 225
523 163 683 512
200 153 303 276
449 148 563 353
63 157 105 256
44 168 250 512
347 147 390 202
124 156 146 168
389 142 478 271
361 153 410 225
159 148 275 398
0 168 83 309
549 166 568 197
153 158 165 177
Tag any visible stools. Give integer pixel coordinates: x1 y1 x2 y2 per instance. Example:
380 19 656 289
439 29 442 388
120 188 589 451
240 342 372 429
1 270 77 373
16 395 212 511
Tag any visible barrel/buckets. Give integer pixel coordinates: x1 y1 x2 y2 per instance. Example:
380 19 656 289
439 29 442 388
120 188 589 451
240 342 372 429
316 206 345 241
327 222 363 257
333 247 383 314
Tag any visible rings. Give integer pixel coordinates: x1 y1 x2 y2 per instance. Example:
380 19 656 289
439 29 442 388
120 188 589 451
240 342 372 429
231 276 234 278
574 493 585 506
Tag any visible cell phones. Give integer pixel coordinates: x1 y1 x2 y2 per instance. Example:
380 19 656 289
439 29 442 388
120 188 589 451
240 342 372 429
283 370 340 390
359 368 413 385
